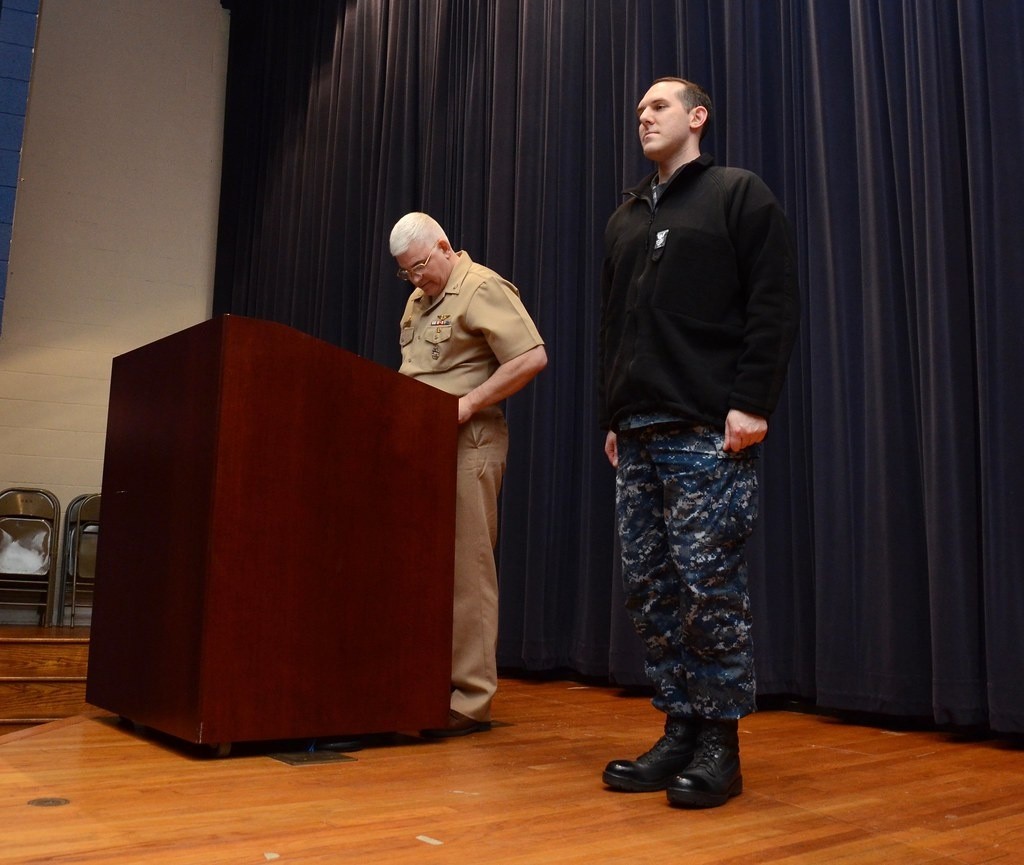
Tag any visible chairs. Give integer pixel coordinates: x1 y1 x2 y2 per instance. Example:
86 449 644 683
58 491 101 628
0 487 60 626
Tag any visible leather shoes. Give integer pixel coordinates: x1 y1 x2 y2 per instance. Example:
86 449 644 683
420 709 491 737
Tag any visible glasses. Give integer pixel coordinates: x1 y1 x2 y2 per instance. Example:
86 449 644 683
397 241 439 281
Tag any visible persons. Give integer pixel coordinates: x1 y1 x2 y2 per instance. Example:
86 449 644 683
390 212 548 738
598 77 801 809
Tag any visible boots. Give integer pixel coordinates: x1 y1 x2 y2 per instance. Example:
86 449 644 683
667 719 743 808
602 715 701 791
